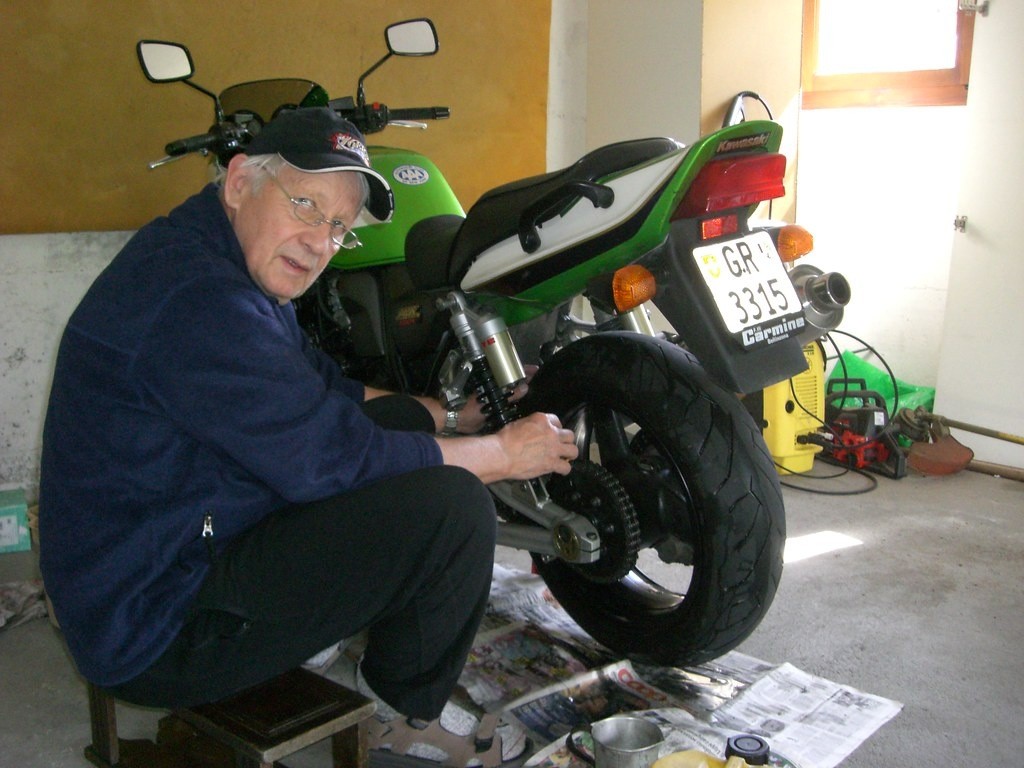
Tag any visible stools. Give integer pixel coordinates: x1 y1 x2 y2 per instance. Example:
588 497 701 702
83 663 377 768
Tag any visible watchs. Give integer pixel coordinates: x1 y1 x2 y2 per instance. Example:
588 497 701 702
442 410 459 436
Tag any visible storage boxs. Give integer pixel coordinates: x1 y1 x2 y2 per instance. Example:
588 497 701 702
0 486 35 554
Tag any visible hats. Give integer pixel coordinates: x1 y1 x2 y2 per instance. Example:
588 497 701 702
265 106 395 223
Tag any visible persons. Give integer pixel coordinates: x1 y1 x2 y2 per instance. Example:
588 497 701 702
36 105 580 717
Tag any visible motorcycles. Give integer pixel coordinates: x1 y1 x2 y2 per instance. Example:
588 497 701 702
131 16 853 673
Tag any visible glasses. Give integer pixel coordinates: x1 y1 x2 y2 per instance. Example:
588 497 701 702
251 161 363 250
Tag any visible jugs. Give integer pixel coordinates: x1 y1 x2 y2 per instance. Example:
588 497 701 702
567 717 665 768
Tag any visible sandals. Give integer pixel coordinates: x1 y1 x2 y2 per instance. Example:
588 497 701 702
353 663 535 768
300 635 355 676
